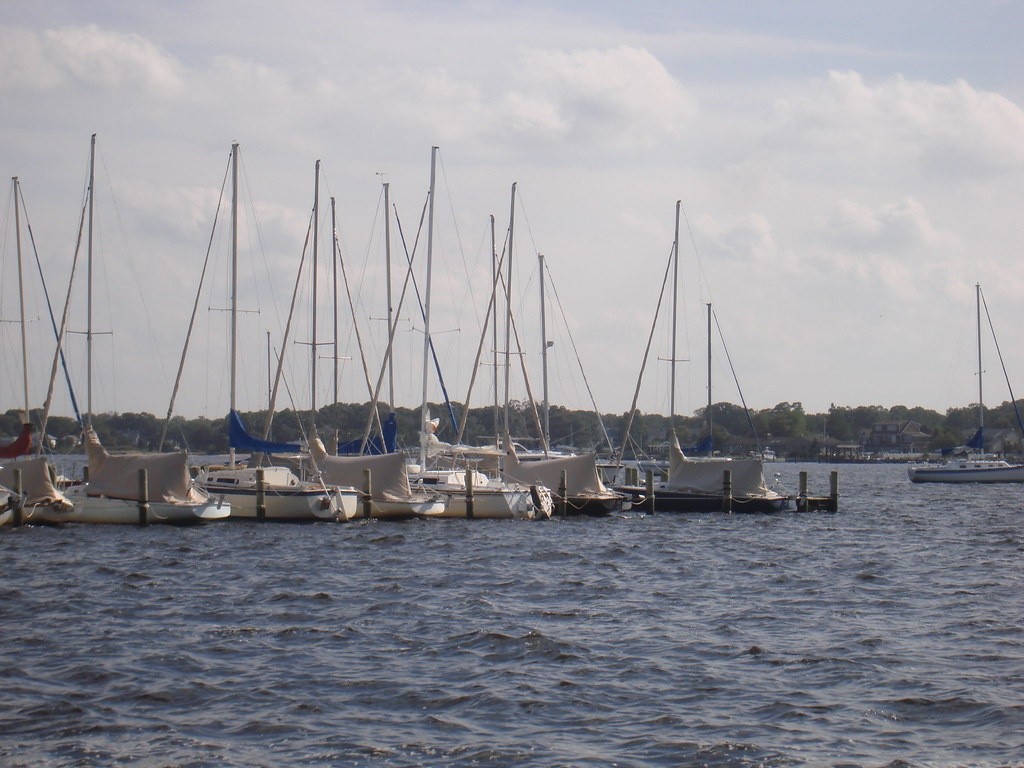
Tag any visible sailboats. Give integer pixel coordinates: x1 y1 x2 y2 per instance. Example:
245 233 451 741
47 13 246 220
907 280 1024 483
0 130 802 528
